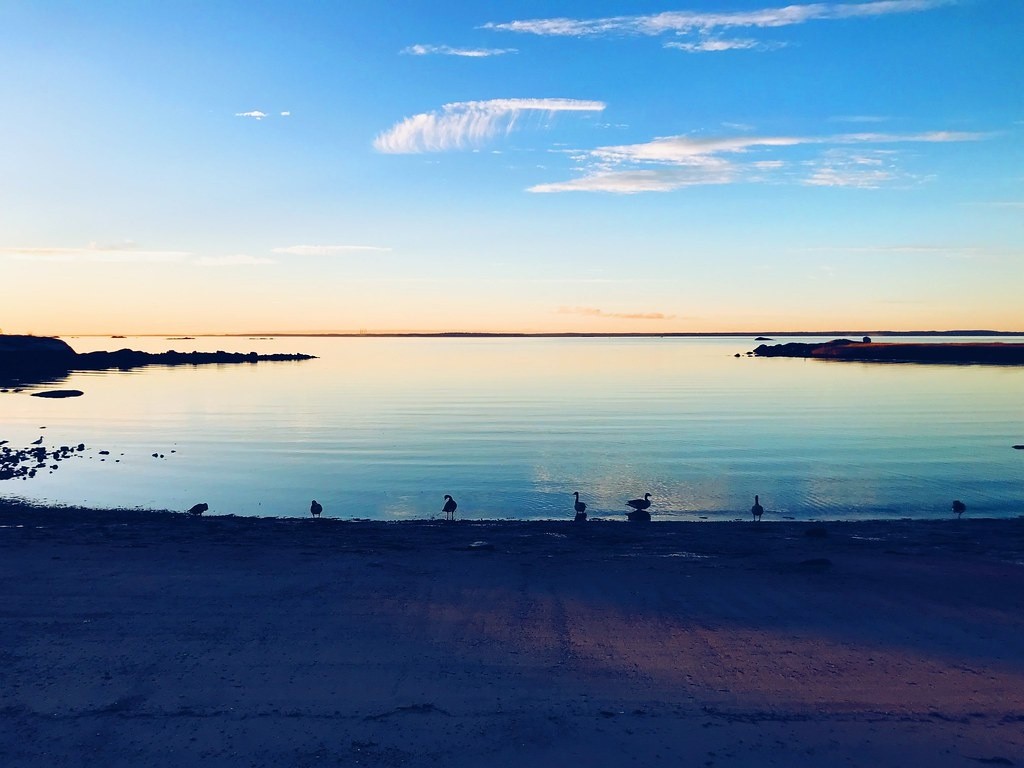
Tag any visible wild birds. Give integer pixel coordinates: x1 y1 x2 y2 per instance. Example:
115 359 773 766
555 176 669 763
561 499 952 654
441 495 457 520
310 500 323 518
624 492 652 511
30 436 45 447
952 500 966 519
572 491 588 514
751 495 763 522
187 502 209 517
